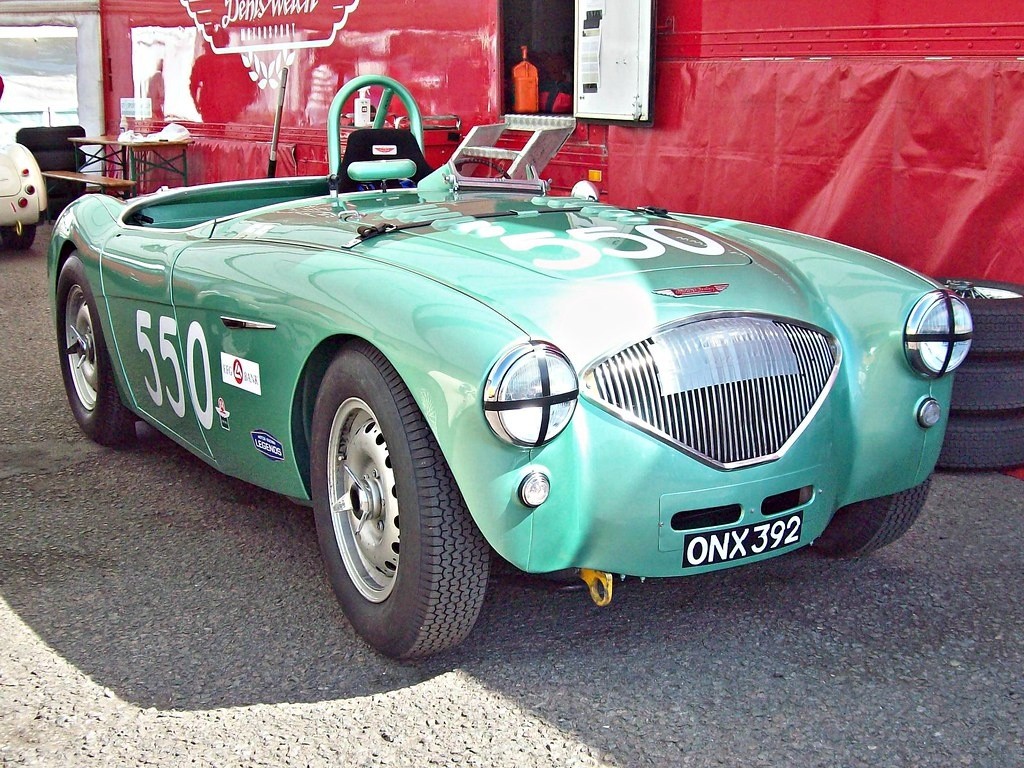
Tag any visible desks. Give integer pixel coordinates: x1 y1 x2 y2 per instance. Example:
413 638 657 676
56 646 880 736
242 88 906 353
67 136 196 200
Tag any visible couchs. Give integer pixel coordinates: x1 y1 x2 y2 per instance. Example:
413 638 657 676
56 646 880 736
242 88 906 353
15 125 86 219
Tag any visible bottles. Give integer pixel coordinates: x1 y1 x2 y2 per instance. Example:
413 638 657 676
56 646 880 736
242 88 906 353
120 115 128 133
512 46 539 113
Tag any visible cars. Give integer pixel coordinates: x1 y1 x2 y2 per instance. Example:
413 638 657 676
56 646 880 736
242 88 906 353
0 74 48 250
46 74 975 662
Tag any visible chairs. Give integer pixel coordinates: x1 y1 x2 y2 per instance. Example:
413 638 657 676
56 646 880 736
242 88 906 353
335 129 433 193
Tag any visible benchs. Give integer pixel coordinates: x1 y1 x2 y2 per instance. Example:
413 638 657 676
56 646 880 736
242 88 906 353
42 171 137 225
